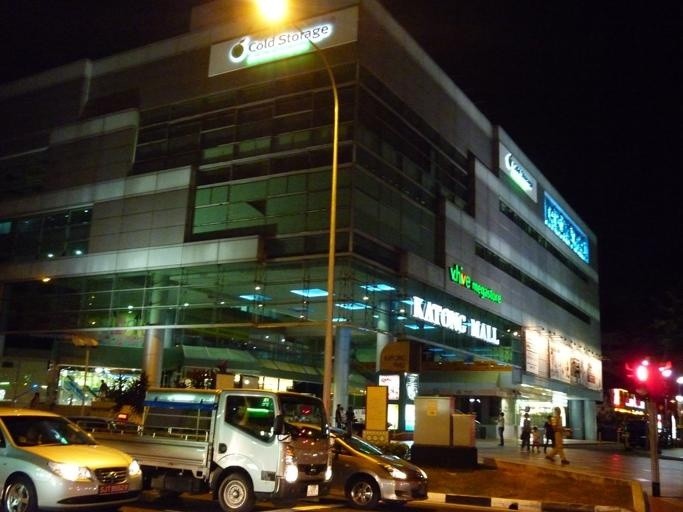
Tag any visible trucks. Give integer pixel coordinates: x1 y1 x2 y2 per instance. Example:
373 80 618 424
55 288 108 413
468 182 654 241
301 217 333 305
69 385 332 512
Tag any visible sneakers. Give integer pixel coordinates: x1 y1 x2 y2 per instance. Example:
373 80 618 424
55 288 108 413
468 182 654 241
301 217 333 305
497 443 570 464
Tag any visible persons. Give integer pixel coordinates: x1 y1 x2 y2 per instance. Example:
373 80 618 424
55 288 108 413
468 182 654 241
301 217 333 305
496 413 504 446
520 406 570 464
97 380 110 396
30 390 58 409
336 404 354 435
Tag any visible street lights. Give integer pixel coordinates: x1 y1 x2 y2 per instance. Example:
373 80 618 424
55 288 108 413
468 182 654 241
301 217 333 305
250 0 341 431
625 349 675 498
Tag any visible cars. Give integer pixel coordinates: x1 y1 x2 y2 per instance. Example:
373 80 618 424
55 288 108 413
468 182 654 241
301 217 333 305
0 408 146 511
69 415 139 430
327 426 429 510
